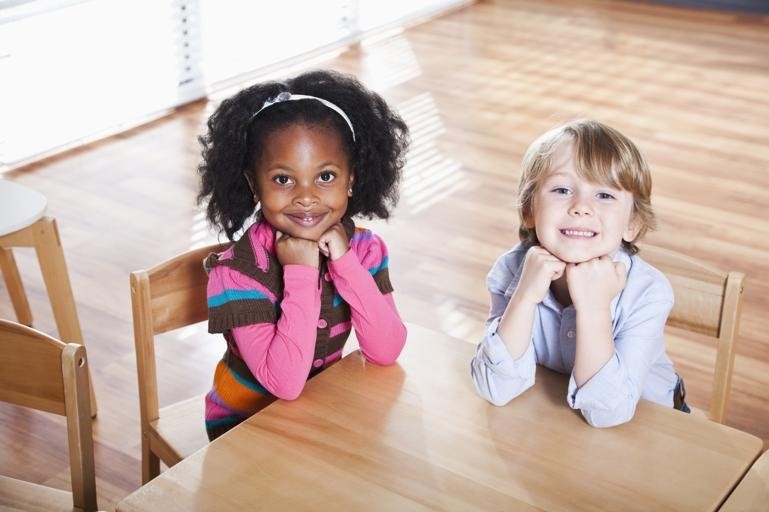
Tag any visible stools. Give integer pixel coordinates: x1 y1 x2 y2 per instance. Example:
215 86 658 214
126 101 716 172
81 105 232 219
1 179 97 417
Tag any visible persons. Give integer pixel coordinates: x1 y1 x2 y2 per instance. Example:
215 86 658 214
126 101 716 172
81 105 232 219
470 118 691 429
195 69 407 442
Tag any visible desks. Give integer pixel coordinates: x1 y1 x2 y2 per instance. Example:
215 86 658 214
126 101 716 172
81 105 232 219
116 316 764 512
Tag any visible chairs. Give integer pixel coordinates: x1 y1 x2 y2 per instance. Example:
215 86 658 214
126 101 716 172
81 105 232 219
0 318 130 509
129 236 243 491
627 235 743 429
